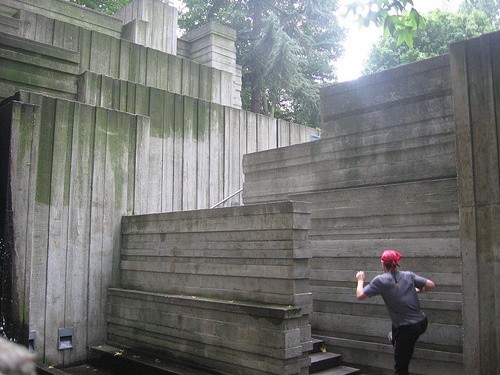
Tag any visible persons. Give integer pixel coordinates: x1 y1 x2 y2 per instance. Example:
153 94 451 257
356 249 434 375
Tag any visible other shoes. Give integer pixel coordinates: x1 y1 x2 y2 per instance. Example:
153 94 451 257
388 331 392 342
